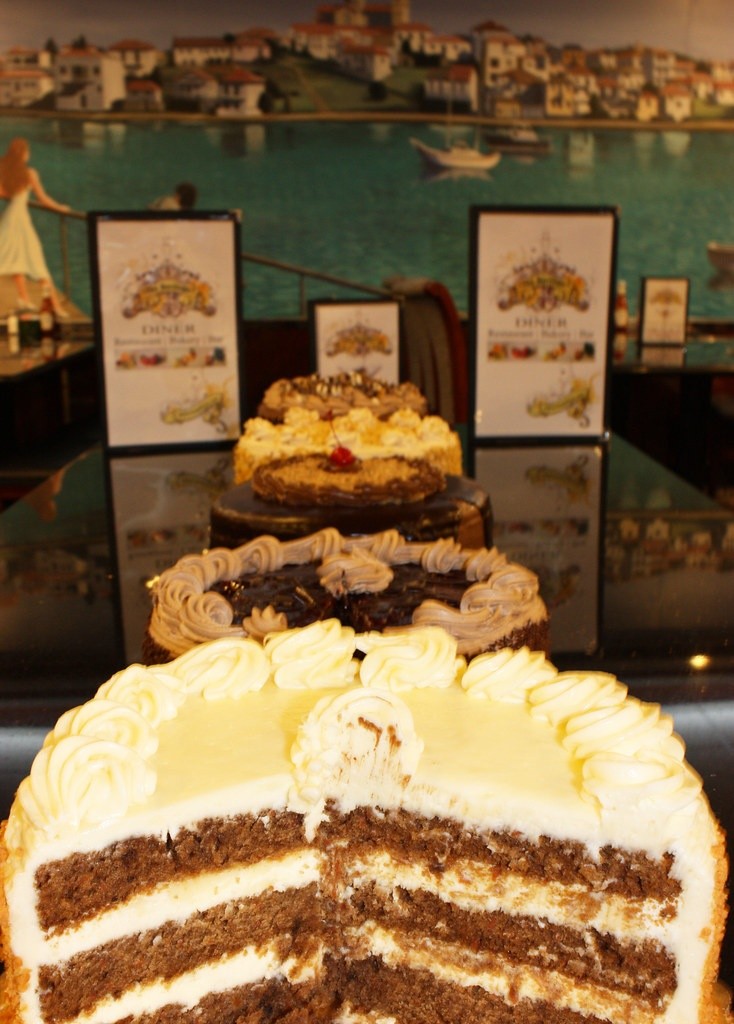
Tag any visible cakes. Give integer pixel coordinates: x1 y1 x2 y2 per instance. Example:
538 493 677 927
1 618 732 1022
145 528 554 669
234 369 462 503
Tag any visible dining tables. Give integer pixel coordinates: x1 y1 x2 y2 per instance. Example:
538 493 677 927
0 424 733 1013
608 331 734 412
